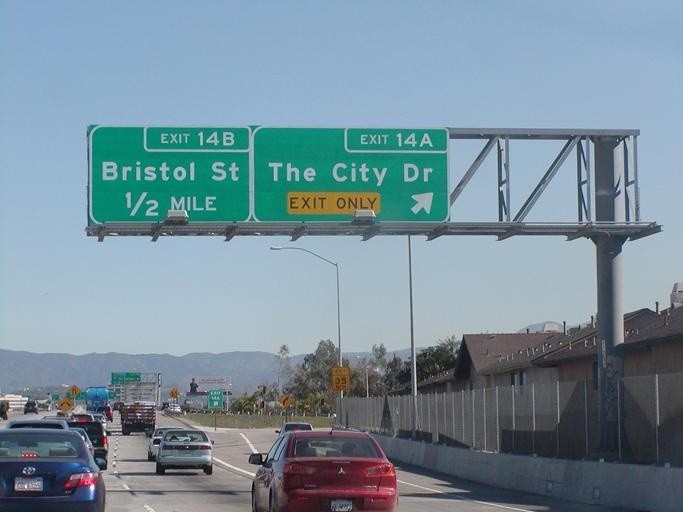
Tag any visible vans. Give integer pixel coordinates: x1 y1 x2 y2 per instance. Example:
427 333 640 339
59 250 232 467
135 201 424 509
274 421 314 446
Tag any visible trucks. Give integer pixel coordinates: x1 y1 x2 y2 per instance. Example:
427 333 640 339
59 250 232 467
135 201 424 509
85 381 160 437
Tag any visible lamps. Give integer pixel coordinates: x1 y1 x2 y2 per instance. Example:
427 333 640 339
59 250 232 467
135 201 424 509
165 210 189 222
352 210 376 223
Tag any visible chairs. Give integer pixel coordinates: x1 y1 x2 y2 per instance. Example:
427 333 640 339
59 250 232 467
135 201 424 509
49 447 71 457
297 443 366 456
0 448 9 456
170 439 201 442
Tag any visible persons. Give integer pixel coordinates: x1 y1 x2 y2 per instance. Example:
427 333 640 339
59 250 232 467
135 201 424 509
190 378 199 392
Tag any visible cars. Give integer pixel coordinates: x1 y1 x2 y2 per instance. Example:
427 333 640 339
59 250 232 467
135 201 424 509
0 400 216 512
246 428 398 512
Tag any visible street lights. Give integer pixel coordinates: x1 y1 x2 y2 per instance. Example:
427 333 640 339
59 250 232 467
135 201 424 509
270 245 343 431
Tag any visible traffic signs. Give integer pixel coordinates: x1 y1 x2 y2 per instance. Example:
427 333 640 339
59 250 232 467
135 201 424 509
251 128 450 227
331 367 349 390
109 372 141 384
86 125 251 225
